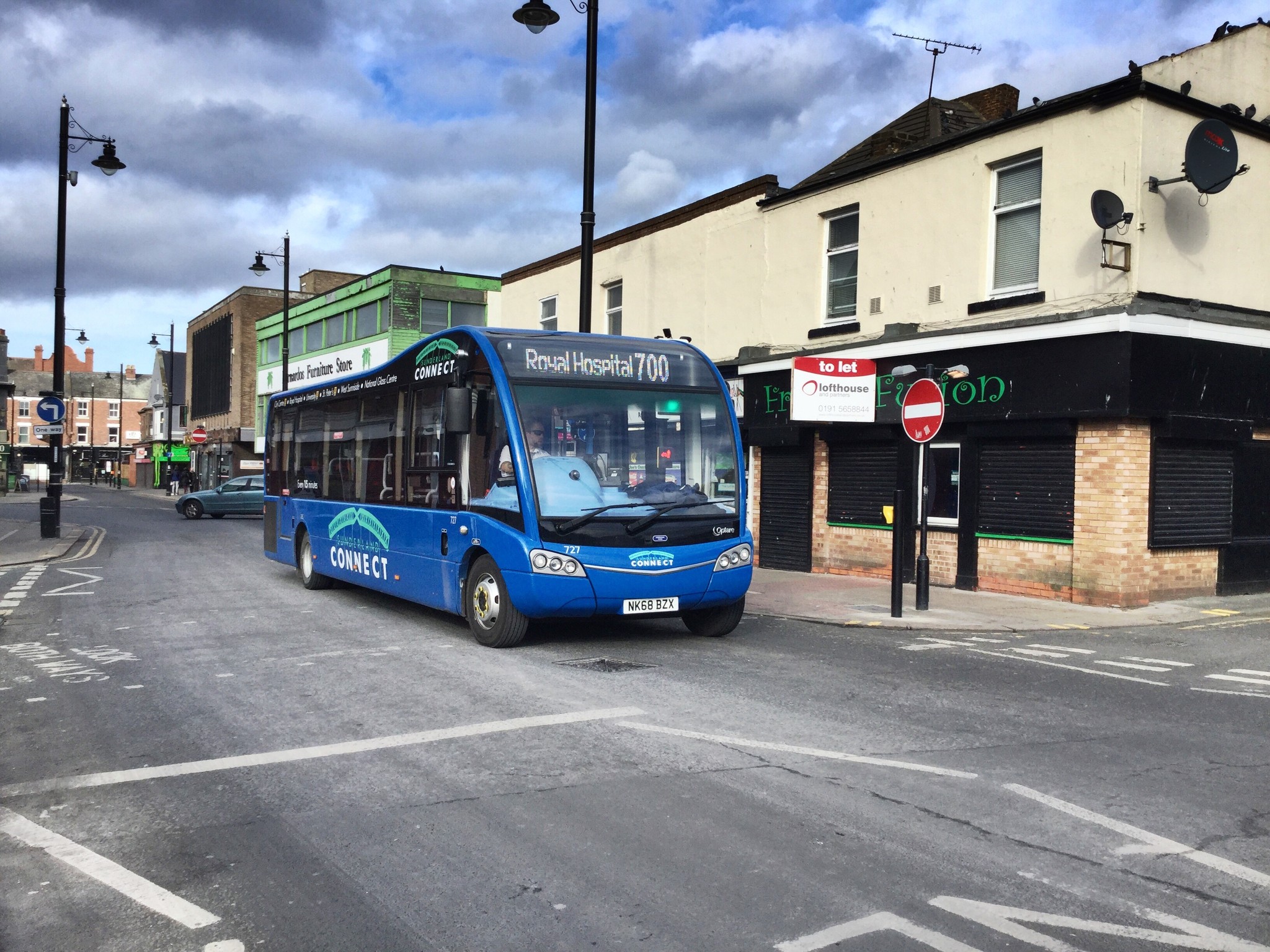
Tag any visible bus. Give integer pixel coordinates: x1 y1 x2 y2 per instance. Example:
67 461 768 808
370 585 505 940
263 325 755 650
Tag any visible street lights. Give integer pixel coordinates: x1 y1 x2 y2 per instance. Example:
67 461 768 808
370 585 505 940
38 93 131 529
890 364 973 619
77 382 94 485
247 232 290 391
102 363 123 489
510 0 598 334
147 320 174 498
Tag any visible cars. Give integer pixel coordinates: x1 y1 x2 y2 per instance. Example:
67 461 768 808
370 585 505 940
174 474 268 520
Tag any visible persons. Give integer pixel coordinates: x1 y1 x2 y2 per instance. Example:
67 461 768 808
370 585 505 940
181 467 197 495
499 419 553 478
170 465 181 495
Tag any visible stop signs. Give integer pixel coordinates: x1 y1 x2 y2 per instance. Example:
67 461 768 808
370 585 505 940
901 378 944 444
193 428 208 443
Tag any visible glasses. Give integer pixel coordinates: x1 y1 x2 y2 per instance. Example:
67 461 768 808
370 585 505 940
531 430 545 436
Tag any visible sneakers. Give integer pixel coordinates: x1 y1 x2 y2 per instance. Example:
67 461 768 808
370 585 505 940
170 493 174 495
175 493 178 495
